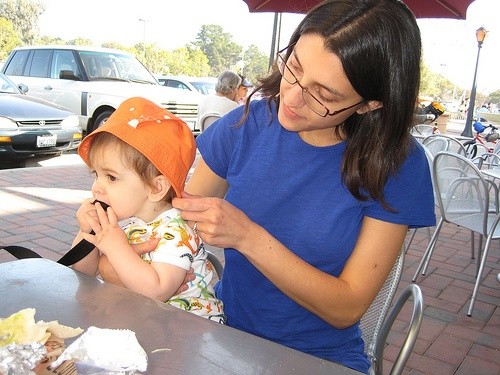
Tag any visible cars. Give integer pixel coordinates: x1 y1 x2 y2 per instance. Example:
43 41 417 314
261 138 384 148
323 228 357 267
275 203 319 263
0 71 83 169
157 76 207 95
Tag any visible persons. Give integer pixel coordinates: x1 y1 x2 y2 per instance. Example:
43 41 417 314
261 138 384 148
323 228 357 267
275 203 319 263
232 73 253 105
71 96 228 325
197 70 241 128
98 0 437 375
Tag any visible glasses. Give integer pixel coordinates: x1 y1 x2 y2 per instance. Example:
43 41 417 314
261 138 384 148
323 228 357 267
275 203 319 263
276 41 366 117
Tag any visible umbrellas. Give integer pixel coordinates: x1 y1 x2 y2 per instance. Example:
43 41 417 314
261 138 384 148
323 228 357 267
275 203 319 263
243 0 475 20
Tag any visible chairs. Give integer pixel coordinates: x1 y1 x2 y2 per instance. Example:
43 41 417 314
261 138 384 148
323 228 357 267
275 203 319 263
359 124 500 375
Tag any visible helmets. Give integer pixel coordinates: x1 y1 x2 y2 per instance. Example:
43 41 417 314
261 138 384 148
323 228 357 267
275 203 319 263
486 133 499 142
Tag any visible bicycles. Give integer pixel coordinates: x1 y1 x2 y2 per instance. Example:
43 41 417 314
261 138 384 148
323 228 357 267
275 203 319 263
457 115 499 163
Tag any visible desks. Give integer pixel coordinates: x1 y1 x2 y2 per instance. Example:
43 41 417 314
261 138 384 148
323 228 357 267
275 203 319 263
0 257 368 375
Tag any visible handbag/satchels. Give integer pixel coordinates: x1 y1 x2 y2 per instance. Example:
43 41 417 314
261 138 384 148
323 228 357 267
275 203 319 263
473 121 485 133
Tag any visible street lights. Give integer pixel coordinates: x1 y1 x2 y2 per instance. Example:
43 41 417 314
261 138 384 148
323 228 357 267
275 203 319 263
460 26 488 139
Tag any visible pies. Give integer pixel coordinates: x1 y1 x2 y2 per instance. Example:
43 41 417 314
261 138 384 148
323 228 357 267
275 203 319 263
0 306 84 348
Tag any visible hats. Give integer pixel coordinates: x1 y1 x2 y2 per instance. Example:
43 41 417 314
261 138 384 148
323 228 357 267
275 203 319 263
240 77 253 87
78 97 197 198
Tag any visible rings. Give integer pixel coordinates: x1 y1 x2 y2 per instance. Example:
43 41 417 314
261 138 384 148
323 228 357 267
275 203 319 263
194 222 197 230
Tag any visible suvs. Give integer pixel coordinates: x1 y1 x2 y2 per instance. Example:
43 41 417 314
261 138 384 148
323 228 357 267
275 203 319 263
0 43 205 136
